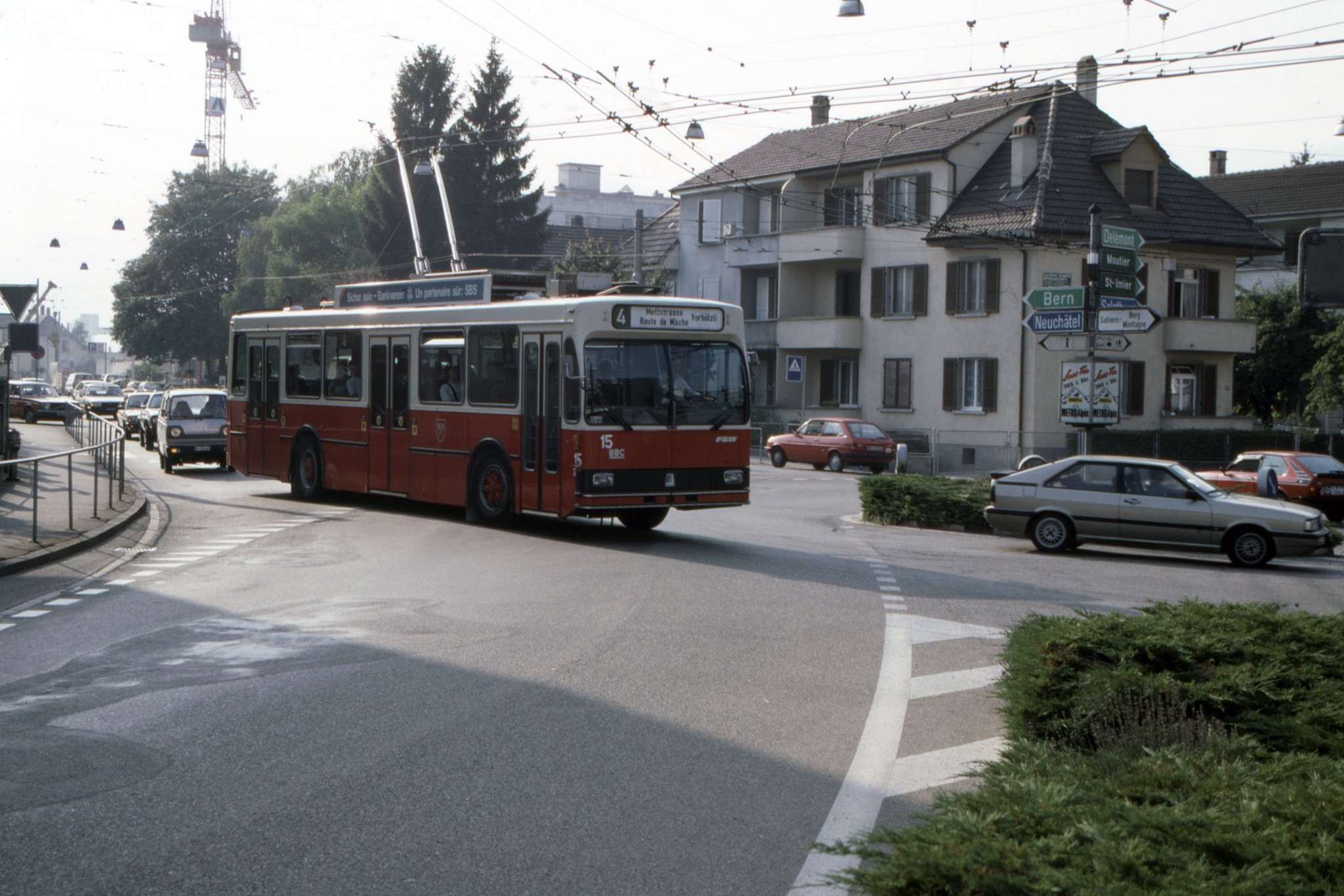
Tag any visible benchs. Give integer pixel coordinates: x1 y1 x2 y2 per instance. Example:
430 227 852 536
1059 474 1084 489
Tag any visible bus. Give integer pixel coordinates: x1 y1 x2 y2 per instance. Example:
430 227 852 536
225 281 760 533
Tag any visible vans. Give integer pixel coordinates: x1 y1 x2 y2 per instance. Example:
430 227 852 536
65 372 94 396
157 387 227 474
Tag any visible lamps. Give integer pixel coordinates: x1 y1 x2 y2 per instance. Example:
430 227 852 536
111 218 126 237
48 238 61 252
414 150 433 177
835 0 865 17
80 262 88 274
190 139 209 157
687 121 704 139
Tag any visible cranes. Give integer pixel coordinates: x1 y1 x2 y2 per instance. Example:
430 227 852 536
188 0 260 175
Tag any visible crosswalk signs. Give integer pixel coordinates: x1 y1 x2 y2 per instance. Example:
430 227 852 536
785 355 804 382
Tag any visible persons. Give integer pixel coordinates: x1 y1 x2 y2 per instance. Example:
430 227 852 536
328 361 348 398
439 360 464 402
1148 469 1186 498
200 395 225 416
346 363 362 400
654 357 713 405
593 360 617 392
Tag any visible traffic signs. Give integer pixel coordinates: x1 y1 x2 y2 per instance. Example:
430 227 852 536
1099 295 1141 309
1095 305 1163 334
1099 248 1144 275
1098 271 1145 297
1020 285 1085 311
1099 224 1146 251
1020 309 1085 334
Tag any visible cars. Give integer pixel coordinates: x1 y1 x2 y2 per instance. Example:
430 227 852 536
8 379 79 425
116 392 151 440
763 417 897 474
1160 449 1344 521
983 454 1331 569
135 392 164 453
73 373 166 430
18 377 56 398
75 382 126 422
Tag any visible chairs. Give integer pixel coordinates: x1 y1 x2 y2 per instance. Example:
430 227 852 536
1125 473 1146 495
596 378 663 406
307 375 329 397
422 368 446 401
